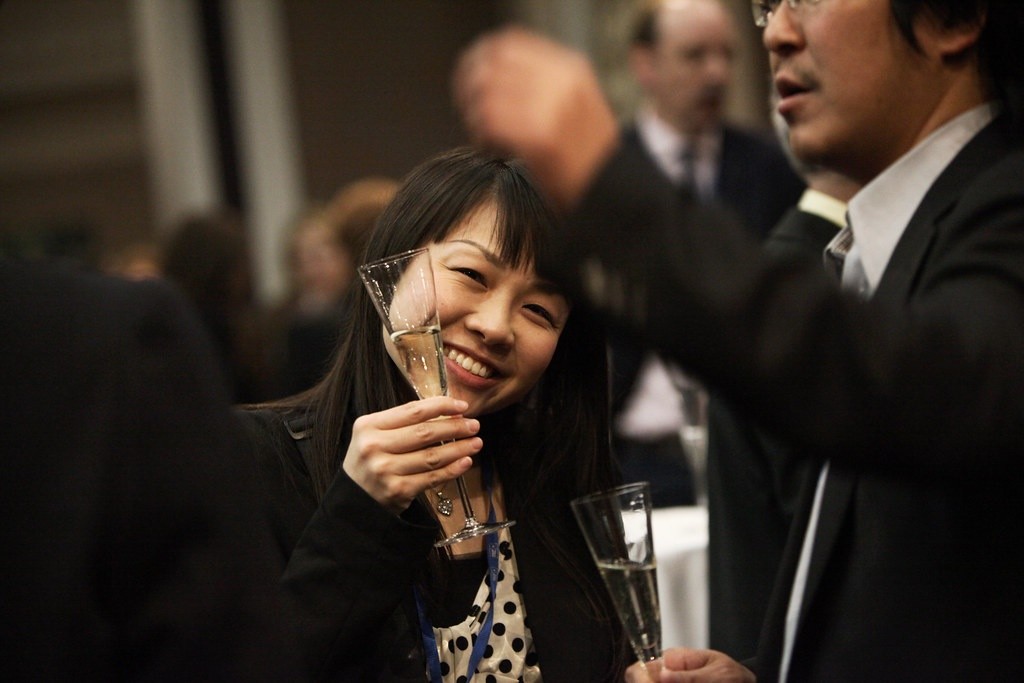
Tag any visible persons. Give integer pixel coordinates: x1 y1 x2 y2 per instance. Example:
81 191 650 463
0 171 407 683
228 147 639 683
442 0 1024 683
623 0 802 235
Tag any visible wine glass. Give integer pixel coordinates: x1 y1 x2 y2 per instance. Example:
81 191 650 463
357 246 515 548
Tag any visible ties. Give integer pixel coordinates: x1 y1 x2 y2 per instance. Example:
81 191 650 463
677 134 701 199
838 240 869 299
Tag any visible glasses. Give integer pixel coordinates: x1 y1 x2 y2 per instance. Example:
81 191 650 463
751 0 805 27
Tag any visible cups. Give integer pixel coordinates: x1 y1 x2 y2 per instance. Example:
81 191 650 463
571 480 667 682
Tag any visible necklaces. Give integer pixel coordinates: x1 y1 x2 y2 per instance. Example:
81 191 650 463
430 482 455 516
